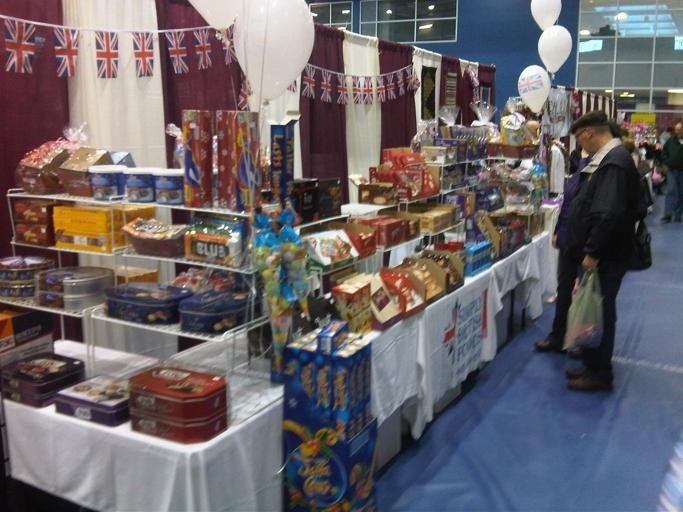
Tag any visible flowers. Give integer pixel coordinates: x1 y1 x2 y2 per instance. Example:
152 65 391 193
621 121 649 144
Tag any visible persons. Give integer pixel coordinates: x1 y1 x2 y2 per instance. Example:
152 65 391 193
535 119 622 355
566 108 644 393
623 119 683 225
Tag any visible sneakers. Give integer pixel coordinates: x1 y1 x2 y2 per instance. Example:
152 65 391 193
647 206 653 214
660 216 682 222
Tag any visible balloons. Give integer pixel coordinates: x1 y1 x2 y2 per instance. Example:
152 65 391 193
531 0 562 32
518 64 550 115
537 24 573 81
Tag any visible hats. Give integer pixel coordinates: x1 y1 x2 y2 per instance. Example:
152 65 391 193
569 109 607 132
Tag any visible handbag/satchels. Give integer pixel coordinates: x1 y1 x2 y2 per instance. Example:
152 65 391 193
628 220 651 270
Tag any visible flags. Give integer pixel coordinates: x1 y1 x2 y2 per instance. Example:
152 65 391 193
288 61 420 105
519 73 543 96
2 17 238 80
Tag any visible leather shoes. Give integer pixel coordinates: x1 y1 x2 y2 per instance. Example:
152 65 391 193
535 339 563 352
565 367 613 390
